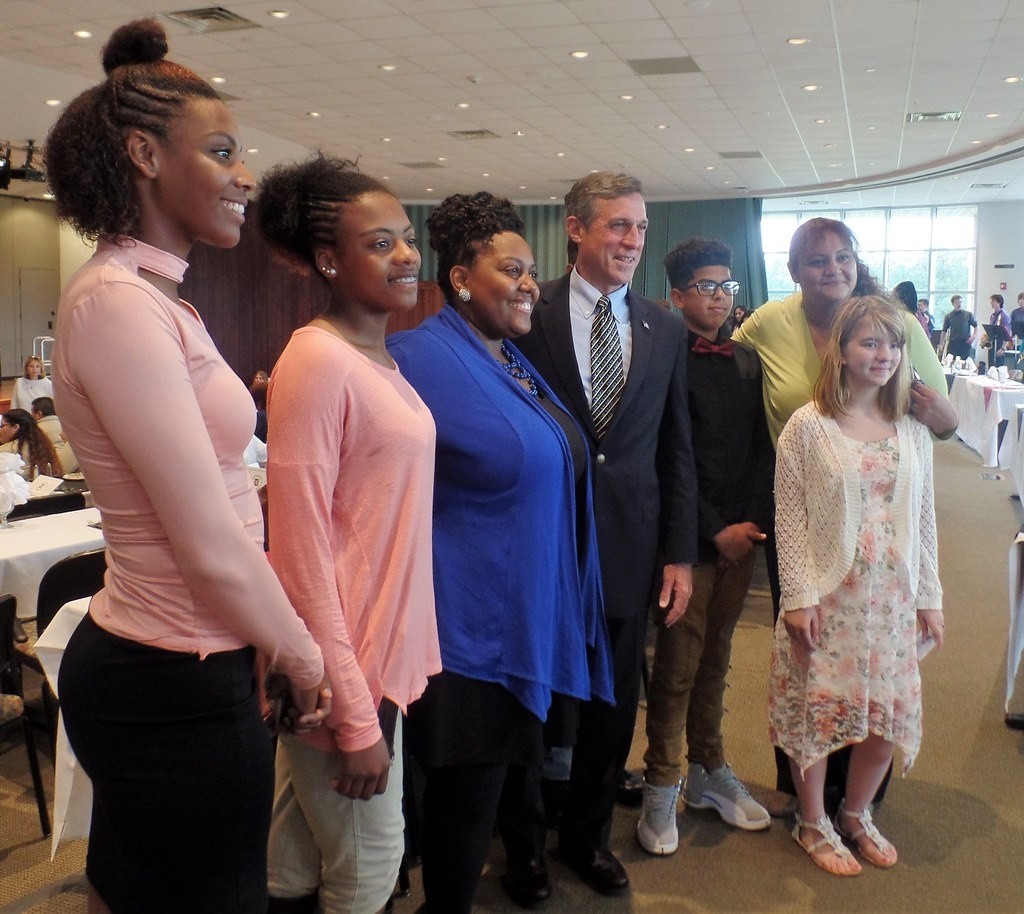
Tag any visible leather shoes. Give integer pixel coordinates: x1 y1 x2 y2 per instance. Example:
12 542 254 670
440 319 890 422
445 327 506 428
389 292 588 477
500 862 552 911
617 770 645 806
576 846 629 897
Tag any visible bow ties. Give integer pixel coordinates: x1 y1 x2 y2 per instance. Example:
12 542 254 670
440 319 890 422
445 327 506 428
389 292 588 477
691 337 737 357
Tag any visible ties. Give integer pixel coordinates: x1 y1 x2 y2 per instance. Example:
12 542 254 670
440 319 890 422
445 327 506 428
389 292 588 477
590 295 624 440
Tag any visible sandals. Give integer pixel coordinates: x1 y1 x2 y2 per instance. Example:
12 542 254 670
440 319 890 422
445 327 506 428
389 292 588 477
834 797 897 868
791 810 862 875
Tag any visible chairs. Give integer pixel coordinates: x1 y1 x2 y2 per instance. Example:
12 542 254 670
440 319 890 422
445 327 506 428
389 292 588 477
1007 369 1023 383
6 491 86 523
929 329 946 365
974 348 989 372
0 546 109 840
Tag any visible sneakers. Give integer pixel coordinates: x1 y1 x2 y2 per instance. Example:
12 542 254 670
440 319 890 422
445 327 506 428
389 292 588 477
637 778 681 854
680 761 771 829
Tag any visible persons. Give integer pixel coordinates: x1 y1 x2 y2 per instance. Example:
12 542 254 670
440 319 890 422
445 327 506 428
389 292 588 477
773 293 942 877
254 370 268 383
731 217 959 821
1010 293 1024 372
242 435 267 469
894 281 930 337
941 295 977 360
981 295 1012 368
919 300 935 333
0 408 63 483
250 382 268 444
11 356 53 414
31 397 80 474
739 310 754 329
731 305 747 332
383 191 616 914
566 237 672 811
636 239 772 853
253 156 441 914
46 20 335 914
502 172 696 903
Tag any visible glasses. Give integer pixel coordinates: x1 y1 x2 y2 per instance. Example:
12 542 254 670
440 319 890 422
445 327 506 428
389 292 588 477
684 279 740 296
954 301 963 305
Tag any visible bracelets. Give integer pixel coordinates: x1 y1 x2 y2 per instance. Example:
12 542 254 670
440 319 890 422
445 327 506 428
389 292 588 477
1001 347 1006 350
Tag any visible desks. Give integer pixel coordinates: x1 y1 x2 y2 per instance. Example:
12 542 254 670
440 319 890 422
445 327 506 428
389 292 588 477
941 364 1024 516
0 507 105 617
